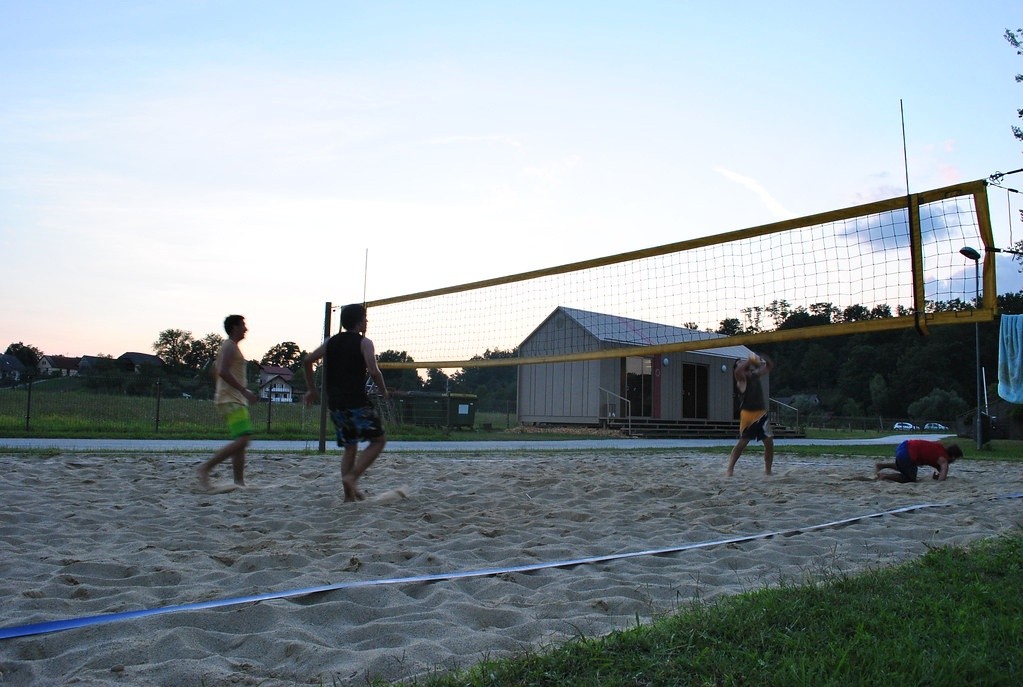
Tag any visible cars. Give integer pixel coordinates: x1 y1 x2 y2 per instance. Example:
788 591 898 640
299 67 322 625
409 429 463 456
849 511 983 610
894 422 920 432
924 423 949 432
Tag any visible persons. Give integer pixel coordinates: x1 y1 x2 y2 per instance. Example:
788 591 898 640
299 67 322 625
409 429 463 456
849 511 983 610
197 315 257 486
876 439 961 483
304 303 390 503
726 353 774 476
